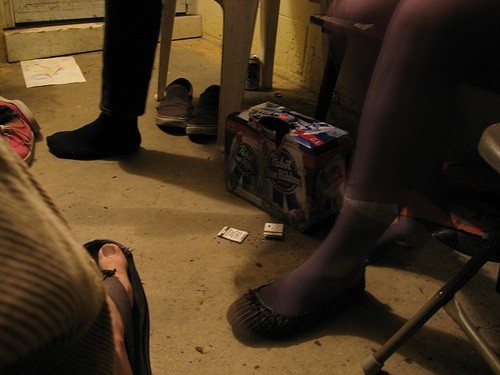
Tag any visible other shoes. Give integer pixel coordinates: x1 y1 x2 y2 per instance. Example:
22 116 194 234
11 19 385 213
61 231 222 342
1 96 40 166
154 78 220 135
227 269 365 339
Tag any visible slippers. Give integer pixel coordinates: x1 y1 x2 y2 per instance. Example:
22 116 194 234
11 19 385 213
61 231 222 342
83 239 151 375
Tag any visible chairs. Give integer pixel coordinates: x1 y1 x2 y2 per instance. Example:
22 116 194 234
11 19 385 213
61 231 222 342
361 126 500 375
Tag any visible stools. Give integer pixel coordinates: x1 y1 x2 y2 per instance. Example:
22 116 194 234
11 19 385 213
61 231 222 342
310 14 433 123
154 0 280 153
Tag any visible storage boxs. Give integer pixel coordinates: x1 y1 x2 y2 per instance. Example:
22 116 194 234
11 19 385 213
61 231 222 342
224 102 349 232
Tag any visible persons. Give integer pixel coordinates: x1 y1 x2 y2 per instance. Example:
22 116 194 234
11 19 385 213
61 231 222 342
0 0 163 374
226 0 500 340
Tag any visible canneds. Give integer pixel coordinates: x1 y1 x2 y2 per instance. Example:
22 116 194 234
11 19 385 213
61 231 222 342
244 55 262 90
